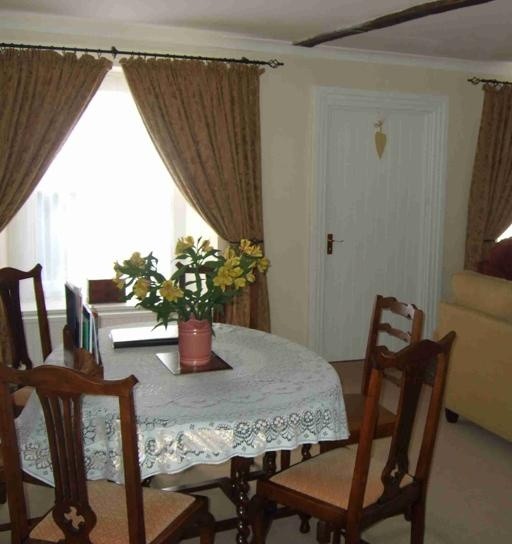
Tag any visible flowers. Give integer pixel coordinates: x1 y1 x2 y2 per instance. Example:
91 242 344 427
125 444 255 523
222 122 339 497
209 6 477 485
114 235 271 337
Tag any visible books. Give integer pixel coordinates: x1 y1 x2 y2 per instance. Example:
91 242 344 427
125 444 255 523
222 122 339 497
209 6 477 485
64 282 101 369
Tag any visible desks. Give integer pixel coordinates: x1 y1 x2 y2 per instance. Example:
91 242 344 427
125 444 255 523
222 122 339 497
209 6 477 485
14 320 349 544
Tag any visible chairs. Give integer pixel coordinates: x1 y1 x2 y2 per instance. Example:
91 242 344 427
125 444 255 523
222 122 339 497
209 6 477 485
249 331 454 542
439 270 512 444
2 364 216 543
317 294 426 455
2 264 54 417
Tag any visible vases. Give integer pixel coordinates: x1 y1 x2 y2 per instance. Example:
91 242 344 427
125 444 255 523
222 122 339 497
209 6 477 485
178 318 213 366
